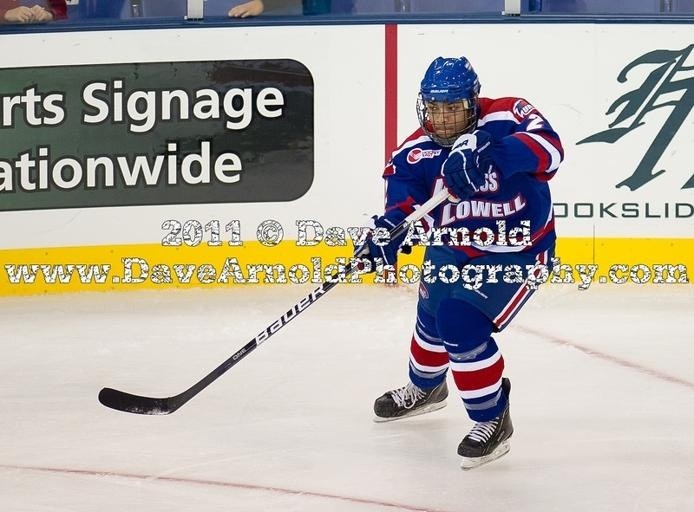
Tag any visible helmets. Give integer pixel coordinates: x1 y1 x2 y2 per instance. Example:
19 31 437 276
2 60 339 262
415 57 481 148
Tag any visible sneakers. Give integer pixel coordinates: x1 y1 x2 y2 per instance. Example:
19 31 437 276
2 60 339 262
374 376 449 418
458 379 512 458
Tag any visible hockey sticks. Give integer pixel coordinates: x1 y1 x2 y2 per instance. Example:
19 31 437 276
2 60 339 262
98 185 449 413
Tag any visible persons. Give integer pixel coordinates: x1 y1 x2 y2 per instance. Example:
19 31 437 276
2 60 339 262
354 56 563 457
229 0 289 18
0 0 68 24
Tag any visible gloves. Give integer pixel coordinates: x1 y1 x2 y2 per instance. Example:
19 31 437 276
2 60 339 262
440 129 505 202
366 207 421 264
